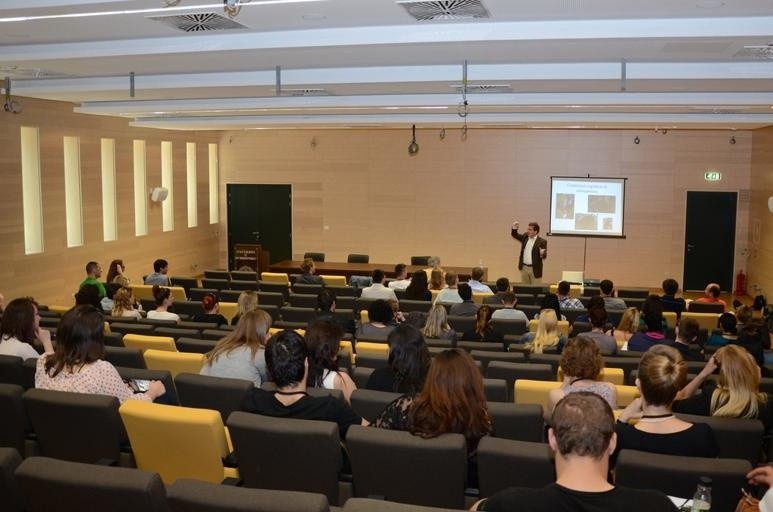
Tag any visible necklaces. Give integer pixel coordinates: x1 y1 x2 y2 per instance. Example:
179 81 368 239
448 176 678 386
274 390 307 396
641 413 674 420
569 378 583 386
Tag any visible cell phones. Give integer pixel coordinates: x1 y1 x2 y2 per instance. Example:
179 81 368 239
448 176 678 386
133 378 152 391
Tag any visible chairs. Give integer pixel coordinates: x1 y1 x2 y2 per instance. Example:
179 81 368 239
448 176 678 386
156 326 195 336
110 364 181 407
514 283 544 294
663 313 676 330
472 293 493 305
166 479 333 512
102 343 146 369
120 399 241 488
230 268 259 278
353 343 391 360
123 333 179 356
174 369 255 412
140 298 172 318
281 306 318 321
347 252 370 263
204 267 231 278
487 360 553 381
361 294 389 311
220 287 286 306
289 292 359 310
346 423 468 511
178 319 218 327
292 283 324 292
517 292 549 306
13 455 168 512
507 342 560 355
679 313 727 335
38 313 64 326
556 363 626 389
480 303 542 324
398 298 434 316
618 287 652 300
232 278 260 291
576 294 595 304
319 307 356 333
201 277 231 290
203 329 237 340
476 435 610 504
143 346 208 378
416 311 480 341
226 409 343 505
469 347 526 363
557 306 630 329
268 326 307 340
176 336 225 353
1 354 24 386
585 287 602 296
330 283 364 294
260 272 291 287
638 407 765 471
171 301 206 319
319 274 349 291
170 274 199 287
515 305 543 321
485 398 543 443
209 300 241 326
437 300 464 315
274 318 310 326
110 319 156 336
456 339 505 353
530 353 642 367
487 316 528 343
514 378 645 427
615 449 755 506
251 302 278 324
706 374 773 391
410 254 433 267
190 287 218 300
128 285 186 299
22 386 136 467
260 380 347 443
353 365 511 404
622 297 648 314
350 273 372 290
305 251 326 262
630 369 703 391
350 390 399 423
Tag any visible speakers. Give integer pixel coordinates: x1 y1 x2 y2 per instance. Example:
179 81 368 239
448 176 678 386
151 188 167 202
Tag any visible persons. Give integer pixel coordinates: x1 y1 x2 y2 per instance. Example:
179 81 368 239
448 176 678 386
423 256 447 284
449 283 480 316
605 307 640 344
354 299 397 343
34 305 167 448
642 293 664 320
429 269 446 290
577 306 617 357
365 325 432 395
595 279 627 310
733 295 767 319
461 305 496 342
669 318 703 361
656 279 686 312
146 284 180 321
100 283 122 312
0 293 4 315
406 270 432 301
575 296 612 326
533 294 567 320
550 335 616 411
466 267 495 295
366 348 496 507
616 344 718 458
231 290 258 326
484 277 515 304
111 287 143 322
694 282 727 305
359 269 398 301
519 308 568 354
433 272 464 307
191 293 228 326
554 281 584 309
627 313 674 350
491 291 530 329
106 259 130 287
706 313 739 348
388 264 412 290
737 304 752 328
746 465 773 512
0 298 56 361
671 344 773 419
303 318 357 405
239 329 370 480
199 309 273 388
299 258 325 285
310 289 356 334
511 221 548 284
79 261 106 297
386 299 407 326
419 305 452 338
144 259 172 287
467 390 681 512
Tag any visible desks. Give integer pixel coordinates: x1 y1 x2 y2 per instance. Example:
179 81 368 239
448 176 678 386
267 260 488 283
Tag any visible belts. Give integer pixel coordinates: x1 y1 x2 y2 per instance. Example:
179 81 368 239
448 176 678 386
522 262 533 267
